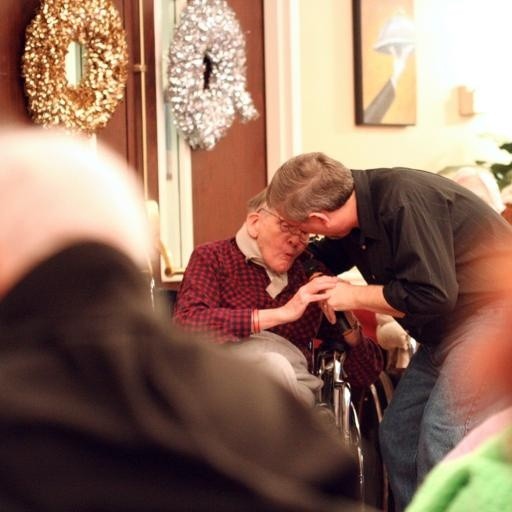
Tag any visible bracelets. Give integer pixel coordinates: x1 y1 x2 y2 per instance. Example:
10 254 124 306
252 303 261 335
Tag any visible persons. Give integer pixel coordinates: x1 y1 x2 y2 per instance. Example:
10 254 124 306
265 153 511 512
171 183 385 391
0 124 367 512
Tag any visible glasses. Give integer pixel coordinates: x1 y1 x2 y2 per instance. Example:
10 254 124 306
257 208 321 244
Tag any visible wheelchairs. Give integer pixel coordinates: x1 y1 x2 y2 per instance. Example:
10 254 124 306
153 289 394 512
